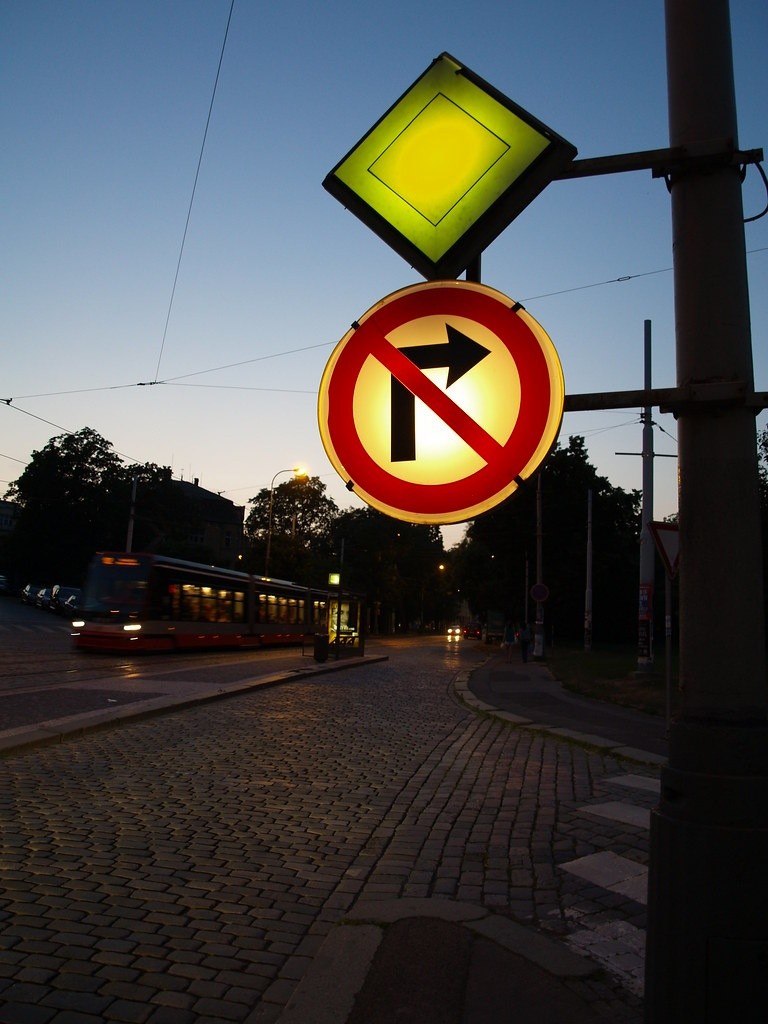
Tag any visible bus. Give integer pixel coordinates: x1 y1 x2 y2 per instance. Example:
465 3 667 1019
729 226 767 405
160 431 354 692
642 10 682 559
71 551 328 650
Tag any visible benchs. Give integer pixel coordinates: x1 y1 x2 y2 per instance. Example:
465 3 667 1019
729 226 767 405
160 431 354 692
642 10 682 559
327 636 360 661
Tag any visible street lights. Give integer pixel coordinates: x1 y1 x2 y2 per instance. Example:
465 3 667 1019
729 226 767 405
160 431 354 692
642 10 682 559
265 468 305 576
421 564 444 623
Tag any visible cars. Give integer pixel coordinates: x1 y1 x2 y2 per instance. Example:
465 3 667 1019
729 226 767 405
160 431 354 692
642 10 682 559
447 625 462 636
464 623 482 639
21 584 80 617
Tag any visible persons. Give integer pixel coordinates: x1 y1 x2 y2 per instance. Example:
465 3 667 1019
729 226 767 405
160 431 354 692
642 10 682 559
502 622 515 664
332 604 349 627
519 622 529 664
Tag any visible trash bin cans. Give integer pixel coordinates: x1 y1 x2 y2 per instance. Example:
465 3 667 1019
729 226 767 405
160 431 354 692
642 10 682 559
314 633 330 663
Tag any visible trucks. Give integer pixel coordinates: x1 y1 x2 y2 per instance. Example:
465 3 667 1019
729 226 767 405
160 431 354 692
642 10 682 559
486 611 505 640
